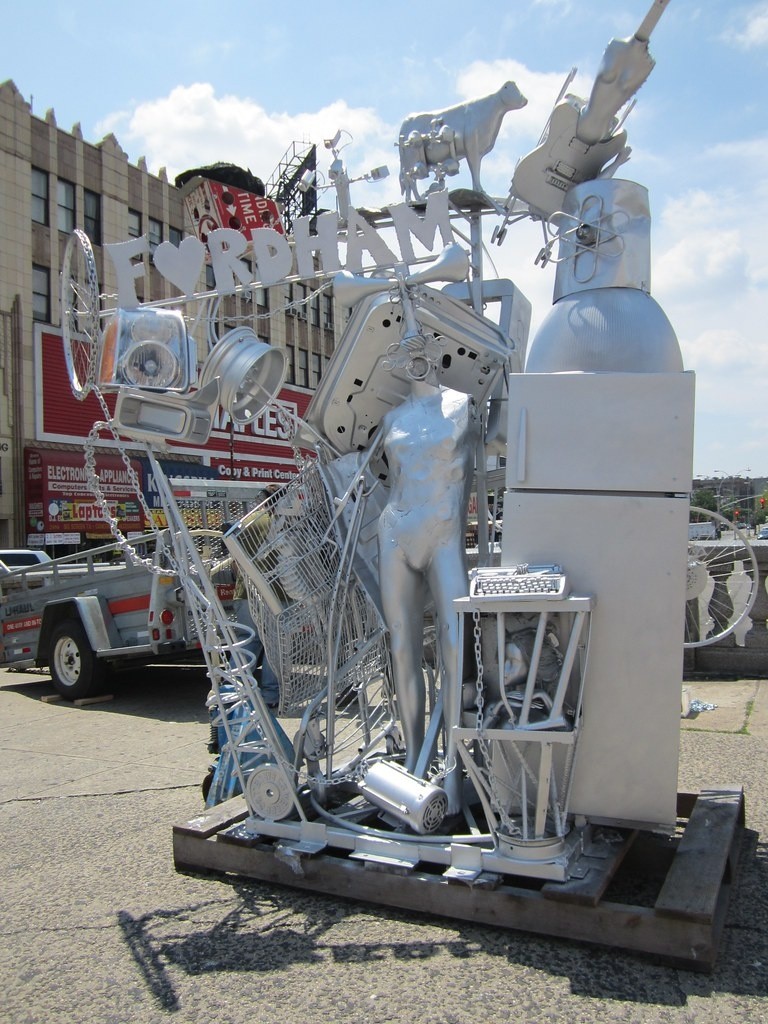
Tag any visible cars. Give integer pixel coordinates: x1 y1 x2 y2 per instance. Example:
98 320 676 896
1 550 51 566
720 523 729 531
756 527 768 540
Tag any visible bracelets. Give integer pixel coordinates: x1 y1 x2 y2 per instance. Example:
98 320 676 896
490 708 499 720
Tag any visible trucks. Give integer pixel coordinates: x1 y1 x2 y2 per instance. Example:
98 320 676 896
0 528 237 701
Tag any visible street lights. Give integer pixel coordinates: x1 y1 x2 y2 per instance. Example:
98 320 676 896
697 475 741 514
715 468 751 540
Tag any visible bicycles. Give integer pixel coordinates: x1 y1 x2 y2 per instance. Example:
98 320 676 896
471 466 758 647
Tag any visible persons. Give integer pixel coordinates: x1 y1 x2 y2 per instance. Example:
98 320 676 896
367 355 480 815
481 631 569 731
224 509 299 709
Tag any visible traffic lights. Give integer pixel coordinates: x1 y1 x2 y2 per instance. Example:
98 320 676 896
760 499 764 509
734 512 739 515
